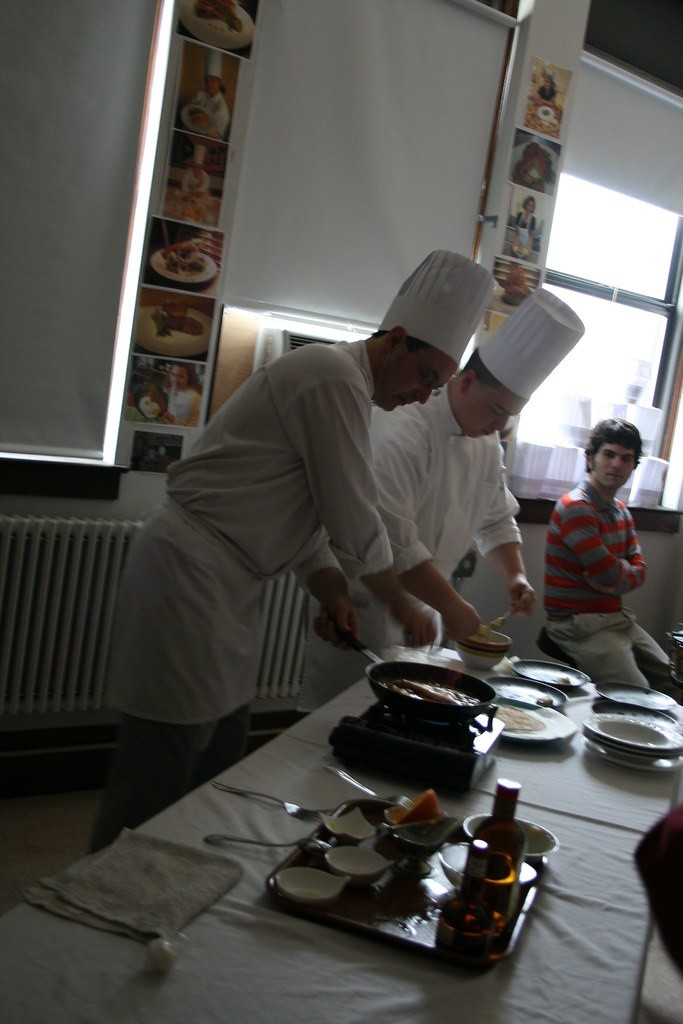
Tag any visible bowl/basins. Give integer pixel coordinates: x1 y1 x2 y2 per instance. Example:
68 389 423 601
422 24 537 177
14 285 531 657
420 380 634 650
512 245 528 258
276 867 350 908
438 846 537 890
463 814 560 864
455 631 513 671
319 807 377 846
324 846 394 885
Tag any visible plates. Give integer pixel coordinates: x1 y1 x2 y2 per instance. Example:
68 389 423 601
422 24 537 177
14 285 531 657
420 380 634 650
486 676 569 708
510 660 591 688
182 106 216 134
149 249 217 282
488 697 577 740
581 714 683 771
509 142 558 196
135 307 212 356
595 682 678 710
179 0 253 49
591 701 679 728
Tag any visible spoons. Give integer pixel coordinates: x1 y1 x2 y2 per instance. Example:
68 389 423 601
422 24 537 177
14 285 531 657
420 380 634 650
205 833 333 857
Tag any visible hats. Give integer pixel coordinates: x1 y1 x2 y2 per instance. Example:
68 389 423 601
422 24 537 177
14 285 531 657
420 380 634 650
477 288 585 400
193 143 207 166
203 48 223 79
378 250 494 365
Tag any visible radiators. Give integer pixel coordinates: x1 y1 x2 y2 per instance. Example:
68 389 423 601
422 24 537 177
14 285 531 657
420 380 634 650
0 510 313 732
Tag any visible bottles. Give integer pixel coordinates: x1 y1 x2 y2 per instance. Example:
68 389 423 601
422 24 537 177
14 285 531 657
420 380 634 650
456 778 526 955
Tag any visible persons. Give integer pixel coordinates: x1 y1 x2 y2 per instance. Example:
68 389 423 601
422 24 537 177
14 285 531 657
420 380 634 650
146 444 172 473
89 248 505 855
181 48 230 194
295 288 585 712
504 264 530 298
633 802 683 971
532 74 557 107
541 418 677 698
155 362 200 426
511 196 536 261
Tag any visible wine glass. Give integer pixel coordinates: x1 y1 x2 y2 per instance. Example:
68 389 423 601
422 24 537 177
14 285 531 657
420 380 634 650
378 816 459 876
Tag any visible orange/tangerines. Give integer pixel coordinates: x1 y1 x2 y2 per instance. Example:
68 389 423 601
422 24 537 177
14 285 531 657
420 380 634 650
397 789 442 826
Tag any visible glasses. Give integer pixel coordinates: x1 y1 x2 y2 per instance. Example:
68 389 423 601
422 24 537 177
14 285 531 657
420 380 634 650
420 377 441 396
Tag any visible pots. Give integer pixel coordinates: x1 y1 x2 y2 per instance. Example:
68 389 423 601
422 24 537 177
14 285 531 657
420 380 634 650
326 616 496 722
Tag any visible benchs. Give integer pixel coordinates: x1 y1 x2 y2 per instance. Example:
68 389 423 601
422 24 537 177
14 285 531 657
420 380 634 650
537 624 683 706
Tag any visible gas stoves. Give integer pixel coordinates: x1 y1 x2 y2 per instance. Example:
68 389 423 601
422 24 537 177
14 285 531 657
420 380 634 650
328 700 506 794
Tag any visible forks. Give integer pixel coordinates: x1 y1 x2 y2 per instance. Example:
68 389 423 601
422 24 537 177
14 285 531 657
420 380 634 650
322 765 412 809
210 780 323 822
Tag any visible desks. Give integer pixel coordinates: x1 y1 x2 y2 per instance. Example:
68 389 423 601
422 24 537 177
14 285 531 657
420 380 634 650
0 641 683 1024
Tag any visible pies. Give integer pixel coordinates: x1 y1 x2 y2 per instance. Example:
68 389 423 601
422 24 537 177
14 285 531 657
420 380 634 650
491 703 548 732
